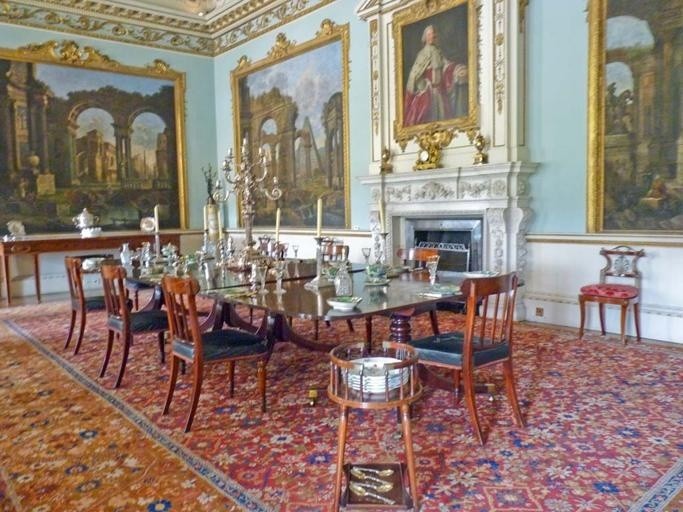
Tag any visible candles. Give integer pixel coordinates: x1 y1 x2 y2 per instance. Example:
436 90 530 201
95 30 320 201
258 146 262 154
275 207 281 243
243 137 246 145
379 199 386 235
228 148 232 156
317 198 323 238
218 210 224 241
154 205 159 233
203 205 207 230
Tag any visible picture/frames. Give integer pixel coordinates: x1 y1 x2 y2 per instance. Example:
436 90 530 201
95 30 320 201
229 19 351 229
390 1 479 151
0 40 189 232
586 0 682 237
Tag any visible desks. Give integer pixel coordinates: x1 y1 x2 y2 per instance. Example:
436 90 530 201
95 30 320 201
99 253 497 423
0 233 182 306
327 340 419 512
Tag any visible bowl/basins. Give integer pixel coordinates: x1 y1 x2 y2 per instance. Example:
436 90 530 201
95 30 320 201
327 297 363 310
84 257 105 266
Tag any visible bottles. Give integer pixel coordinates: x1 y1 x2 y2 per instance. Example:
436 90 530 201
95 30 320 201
71 206 101 231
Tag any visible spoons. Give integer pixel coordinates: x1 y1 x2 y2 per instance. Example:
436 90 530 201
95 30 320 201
348 466 398 505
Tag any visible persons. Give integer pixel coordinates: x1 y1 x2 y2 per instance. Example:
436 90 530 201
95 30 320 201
403 25 469 129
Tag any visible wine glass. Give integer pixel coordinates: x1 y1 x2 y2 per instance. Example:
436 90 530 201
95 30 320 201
116 234 442 296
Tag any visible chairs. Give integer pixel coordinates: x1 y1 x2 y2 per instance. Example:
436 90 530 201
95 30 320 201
95 261 185 389
577 245 645 347
401 271 525 447
64 257 134 356
366 248 440 356
159 272 269 432
313 245 355 341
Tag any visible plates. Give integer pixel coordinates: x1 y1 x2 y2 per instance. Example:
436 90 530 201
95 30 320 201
340 356 410 394
139 216 155 232
